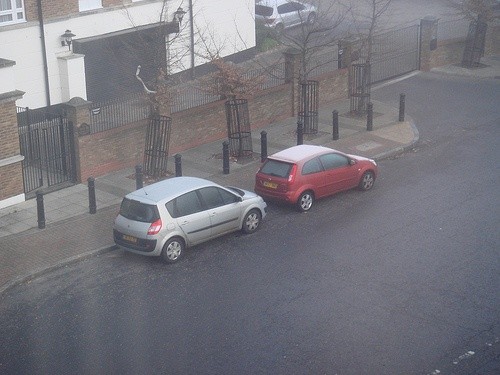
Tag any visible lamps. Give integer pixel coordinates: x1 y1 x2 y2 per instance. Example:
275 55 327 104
174 6 187 27
60 29 76 51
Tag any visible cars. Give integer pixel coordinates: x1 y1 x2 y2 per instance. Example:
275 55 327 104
114 175 267 264
255 144 377 212
256 0 317 34
